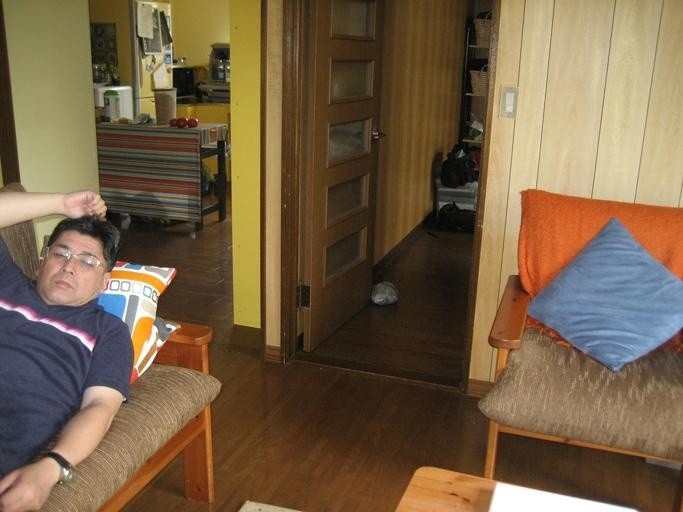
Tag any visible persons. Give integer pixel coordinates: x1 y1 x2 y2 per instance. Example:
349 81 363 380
0 190 134 512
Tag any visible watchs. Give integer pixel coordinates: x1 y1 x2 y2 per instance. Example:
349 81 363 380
41 452 74 482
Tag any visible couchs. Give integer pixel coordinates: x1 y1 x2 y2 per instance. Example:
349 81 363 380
473 185 683 486
2 173 220 512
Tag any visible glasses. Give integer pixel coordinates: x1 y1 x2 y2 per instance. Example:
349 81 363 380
45 246 101 272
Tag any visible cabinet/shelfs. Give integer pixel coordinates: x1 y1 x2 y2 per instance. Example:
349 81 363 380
174 103 232 184
453 8 494 145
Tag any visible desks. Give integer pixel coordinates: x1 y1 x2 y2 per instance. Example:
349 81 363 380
95 115 229 233
391 462 635 512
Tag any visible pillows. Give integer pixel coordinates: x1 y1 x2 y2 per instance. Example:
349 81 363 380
93 254 178 364
127 314 181 385
524 216 683 376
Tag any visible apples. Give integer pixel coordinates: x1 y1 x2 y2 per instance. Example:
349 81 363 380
170 118 199 128
